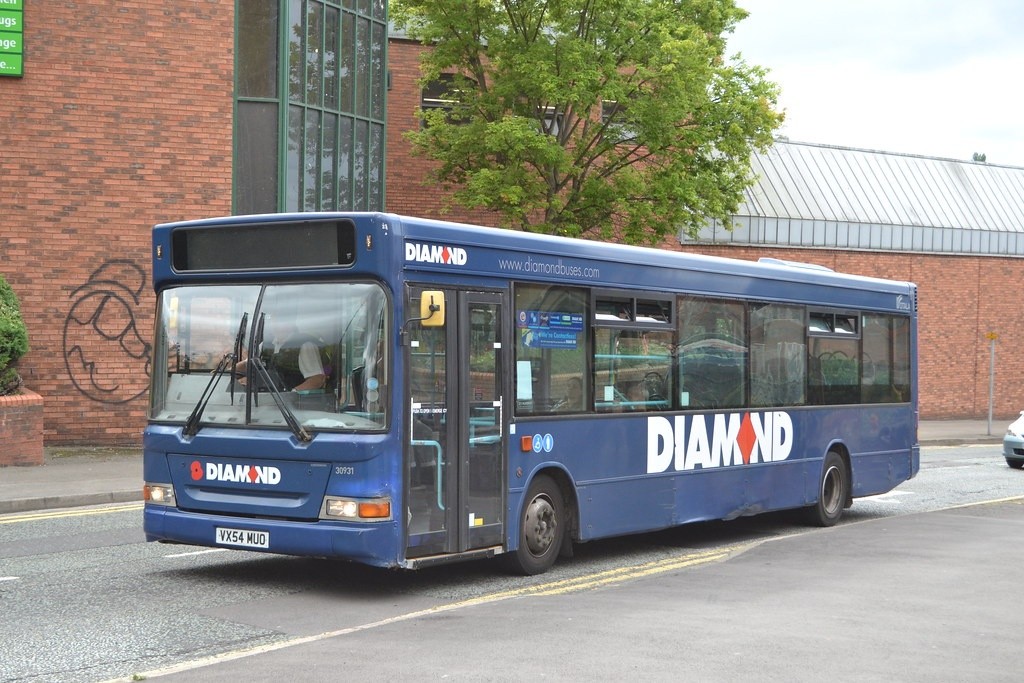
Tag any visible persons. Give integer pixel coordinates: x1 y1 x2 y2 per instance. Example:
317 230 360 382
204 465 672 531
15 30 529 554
552 378 583 413
362 341 436 486
210 300 326 396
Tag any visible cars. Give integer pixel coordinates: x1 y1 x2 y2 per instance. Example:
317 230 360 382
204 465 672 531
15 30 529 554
1003 411 1024 468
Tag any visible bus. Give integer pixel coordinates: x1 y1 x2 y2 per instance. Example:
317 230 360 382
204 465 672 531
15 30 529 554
143 212 920 576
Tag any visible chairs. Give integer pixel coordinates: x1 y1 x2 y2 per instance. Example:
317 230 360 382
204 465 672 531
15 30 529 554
644 352 877 410
